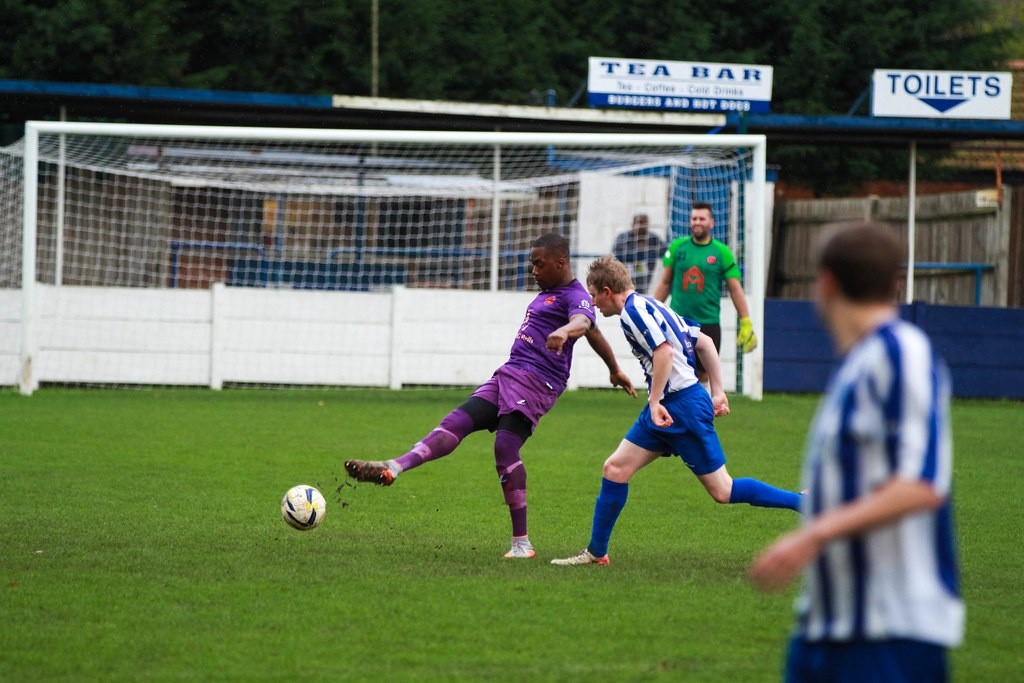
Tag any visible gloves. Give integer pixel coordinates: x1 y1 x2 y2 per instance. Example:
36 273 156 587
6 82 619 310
737 318 758 353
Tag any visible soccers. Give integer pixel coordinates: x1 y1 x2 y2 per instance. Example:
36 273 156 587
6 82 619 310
281 484 328 532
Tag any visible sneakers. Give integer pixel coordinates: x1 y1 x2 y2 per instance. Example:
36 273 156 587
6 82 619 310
501 540 536 559
797 488 810 518
343 458 400 487
550 548 611 566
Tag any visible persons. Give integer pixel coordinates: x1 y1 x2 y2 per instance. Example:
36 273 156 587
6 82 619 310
551 257 808 566
750 222 968 683
344 232 636 558
652 202 758 399
614 214 668 295
